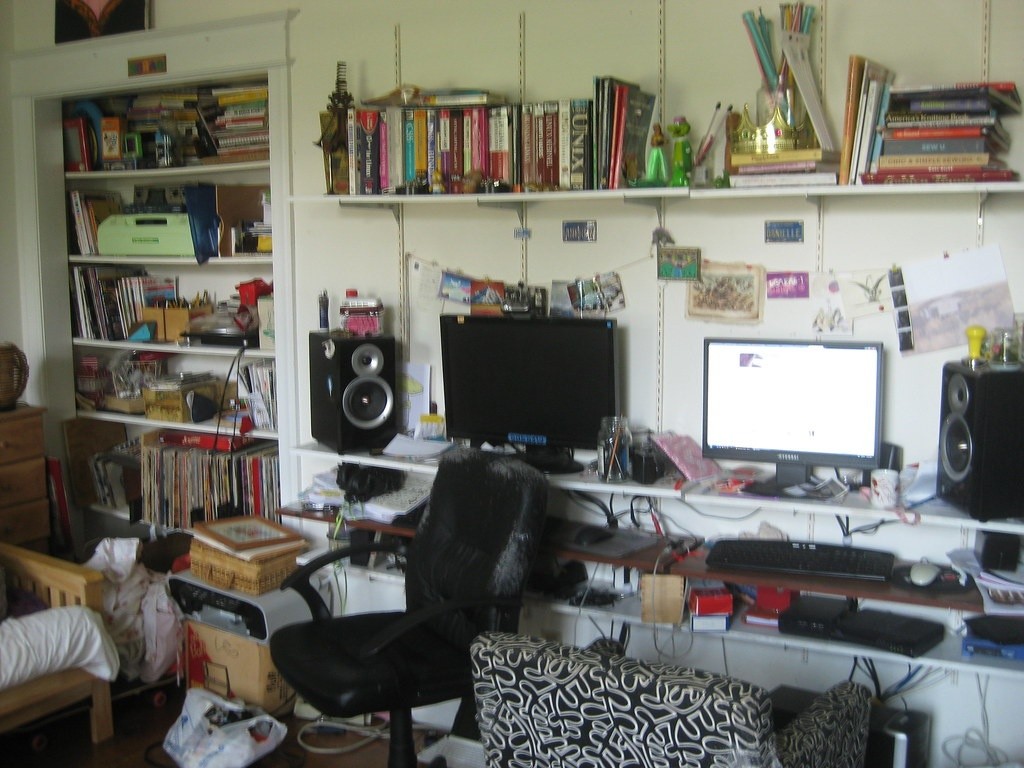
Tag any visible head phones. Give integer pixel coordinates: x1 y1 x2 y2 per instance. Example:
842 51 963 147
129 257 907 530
336 463 404 498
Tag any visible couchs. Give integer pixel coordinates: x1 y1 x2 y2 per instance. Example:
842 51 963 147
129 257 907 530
471 630 873 768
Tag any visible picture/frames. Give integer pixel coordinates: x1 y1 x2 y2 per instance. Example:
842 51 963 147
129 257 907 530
193 513 301 550
55 0 150 44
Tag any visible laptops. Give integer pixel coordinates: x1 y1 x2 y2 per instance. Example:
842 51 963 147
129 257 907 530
417 697 487 768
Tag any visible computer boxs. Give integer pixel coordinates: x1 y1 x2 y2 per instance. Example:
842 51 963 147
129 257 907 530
767 684 930 768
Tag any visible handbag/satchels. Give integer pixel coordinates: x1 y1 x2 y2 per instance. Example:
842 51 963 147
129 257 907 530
163 687 287 768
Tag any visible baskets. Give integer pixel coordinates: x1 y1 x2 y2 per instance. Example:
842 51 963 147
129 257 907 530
189 537 302 596
0 341 30 410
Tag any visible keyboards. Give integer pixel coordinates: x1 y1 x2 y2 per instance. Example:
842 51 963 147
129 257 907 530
706 539 897 582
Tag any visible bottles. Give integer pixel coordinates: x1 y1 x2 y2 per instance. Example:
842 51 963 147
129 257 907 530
596 417 632 483
991 328 1020 365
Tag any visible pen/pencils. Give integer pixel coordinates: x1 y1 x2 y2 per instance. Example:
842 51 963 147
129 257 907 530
604 416 624 480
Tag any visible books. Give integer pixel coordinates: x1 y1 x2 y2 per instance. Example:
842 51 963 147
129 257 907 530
947 547 1024 617
126 84 269 166
68 262 177 341
657 434 720 481
238 357 277 432
741 605 780 627
839 55 1021 185
347 75 656 194
66 189 124 256
86 428 309 562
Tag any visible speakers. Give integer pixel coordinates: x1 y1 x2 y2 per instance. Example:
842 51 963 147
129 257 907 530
863 441 901 487
309 329 397 455
935 360 1024 523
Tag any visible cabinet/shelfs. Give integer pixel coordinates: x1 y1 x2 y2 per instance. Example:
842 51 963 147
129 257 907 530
282 444 1024 688
32 14 272 625
0 404 52 558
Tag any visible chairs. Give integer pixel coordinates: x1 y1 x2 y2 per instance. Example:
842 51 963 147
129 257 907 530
271 448 549 768
0 535 114 768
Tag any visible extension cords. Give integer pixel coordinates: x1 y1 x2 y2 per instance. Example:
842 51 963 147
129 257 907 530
293 697 373 727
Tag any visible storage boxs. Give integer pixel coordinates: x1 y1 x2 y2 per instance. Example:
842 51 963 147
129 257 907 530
172 615 299 714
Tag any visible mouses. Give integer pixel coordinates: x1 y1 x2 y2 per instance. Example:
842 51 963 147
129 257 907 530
573 526 613 546
910 563 941 587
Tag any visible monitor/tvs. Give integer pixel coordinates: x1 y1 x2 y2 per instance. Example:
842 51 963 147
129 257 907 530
440 313 620 474
701 337 883 499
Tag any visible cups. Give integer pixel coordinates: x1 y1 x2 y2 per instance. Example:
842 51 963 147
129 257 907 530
870 469 898 507
755 79 811 131
